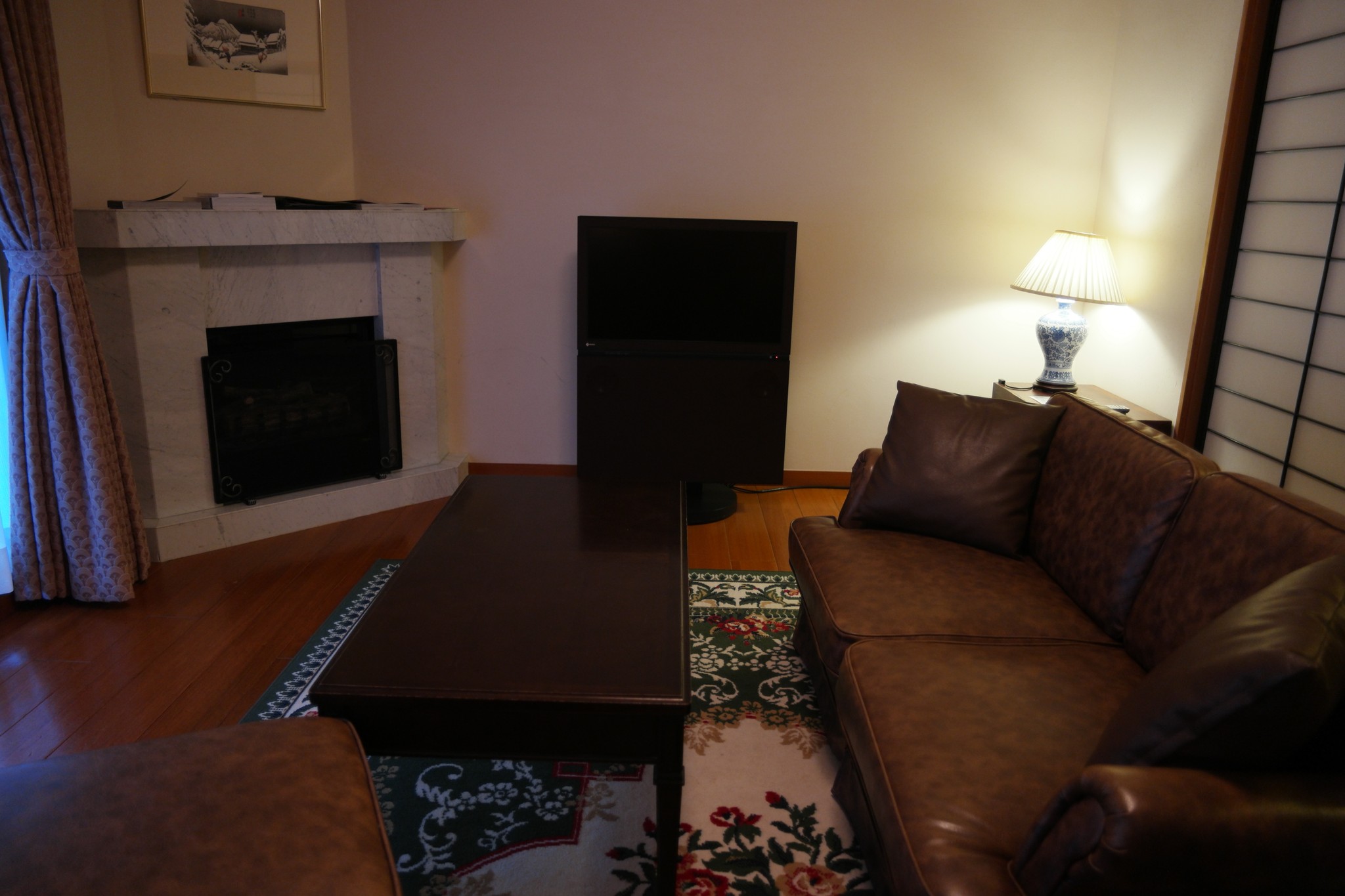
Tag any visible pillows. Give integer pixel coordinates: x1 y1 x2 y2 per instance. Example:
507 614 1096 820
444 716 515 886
1082 549 1345 769
848 379 1068 564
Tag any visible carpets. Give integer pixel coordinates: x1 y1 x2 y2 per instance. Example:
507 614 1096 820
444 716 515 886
237 557 902 896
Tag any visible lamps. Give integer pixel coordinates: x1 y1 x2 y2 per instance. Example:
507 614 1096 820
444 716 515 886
1009 228 1127 398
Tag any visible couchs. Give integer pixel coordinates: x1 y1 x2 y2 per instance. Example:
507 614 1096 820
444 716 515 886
789 392 1345 896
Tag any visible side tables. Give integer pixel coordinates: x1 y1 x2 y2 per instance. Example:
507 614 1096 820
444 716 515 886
993 380 1173 441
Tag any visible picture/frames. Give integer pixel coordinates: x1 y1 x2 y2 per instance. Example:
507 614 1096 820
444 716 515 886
138 0 326 110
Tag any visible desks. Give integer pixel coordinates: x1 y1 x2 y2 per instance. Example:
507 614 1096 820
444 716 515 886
311 474 693 896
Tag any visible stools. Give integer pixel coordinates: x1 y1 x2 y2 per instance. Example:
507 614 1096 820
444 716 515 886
0 713 404 896
